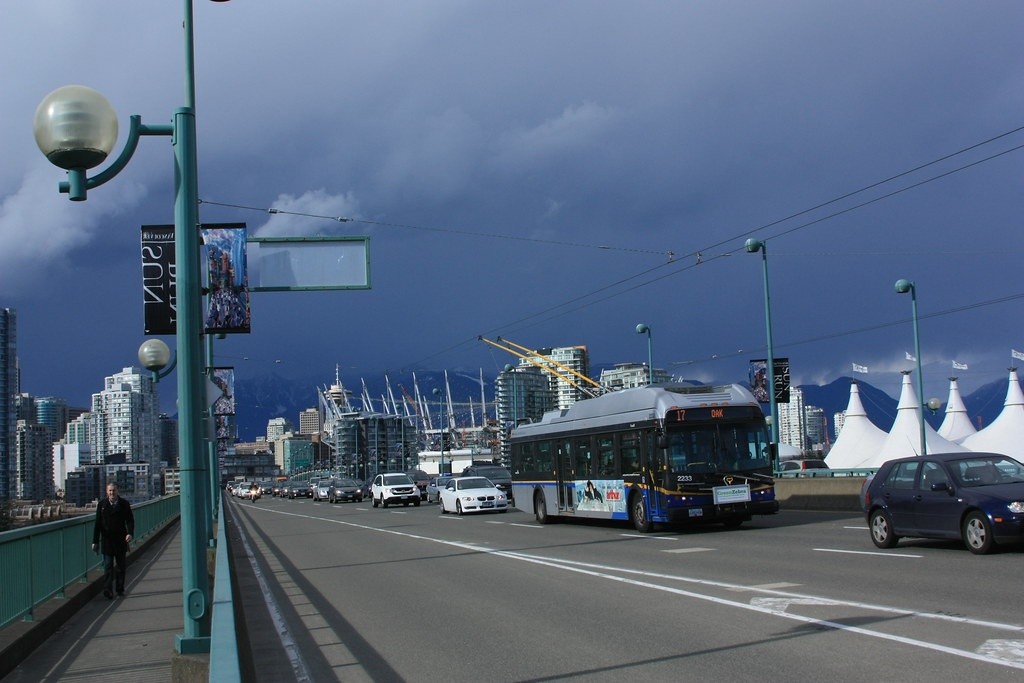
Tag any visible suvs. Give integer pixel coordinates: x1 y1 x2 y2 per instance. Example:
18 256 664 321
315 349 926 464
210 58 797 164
371 473 422 509
407 471 437 499
463 464 513 498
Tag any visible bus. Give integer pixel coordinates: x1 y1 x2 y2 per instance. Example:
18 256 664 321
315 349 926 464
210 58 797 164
475 335 779 533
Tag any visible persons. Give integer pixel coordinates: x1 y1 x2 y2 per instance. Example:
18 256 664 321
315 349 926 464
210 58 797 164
248 483 258 500
585 482 603 503
92 483 135 599
586 458 640 475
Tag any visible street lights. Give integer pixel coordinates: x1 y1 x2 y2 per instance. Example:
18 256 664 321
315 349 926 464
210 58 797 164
139 244 217 547
370 415 379 474
635 322 655 385
351 424 359 480
746 236 784 474
395 404 406 472
33 0 228 651
289 429 344 480
895 279 929 454
433 387 446 477
504 363 519 430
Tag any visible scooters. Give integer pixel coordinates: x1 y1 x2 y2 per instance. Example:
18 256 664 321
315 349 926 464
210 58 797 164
249 488 259 503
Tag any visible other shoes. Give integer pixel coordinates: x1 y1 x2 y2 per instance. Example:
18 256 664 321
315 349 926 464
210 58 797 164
116 585 124 595
104 589 113 600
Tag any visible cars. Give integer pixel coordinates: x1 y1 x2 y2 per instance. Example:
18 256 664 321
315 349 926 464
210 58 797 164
425 476 455 504
328 479 363 503
219 475 321 500
312 481 330 502
441 476 510 514
353 476 376 499
864 453 1024 555
779 459 833 478
